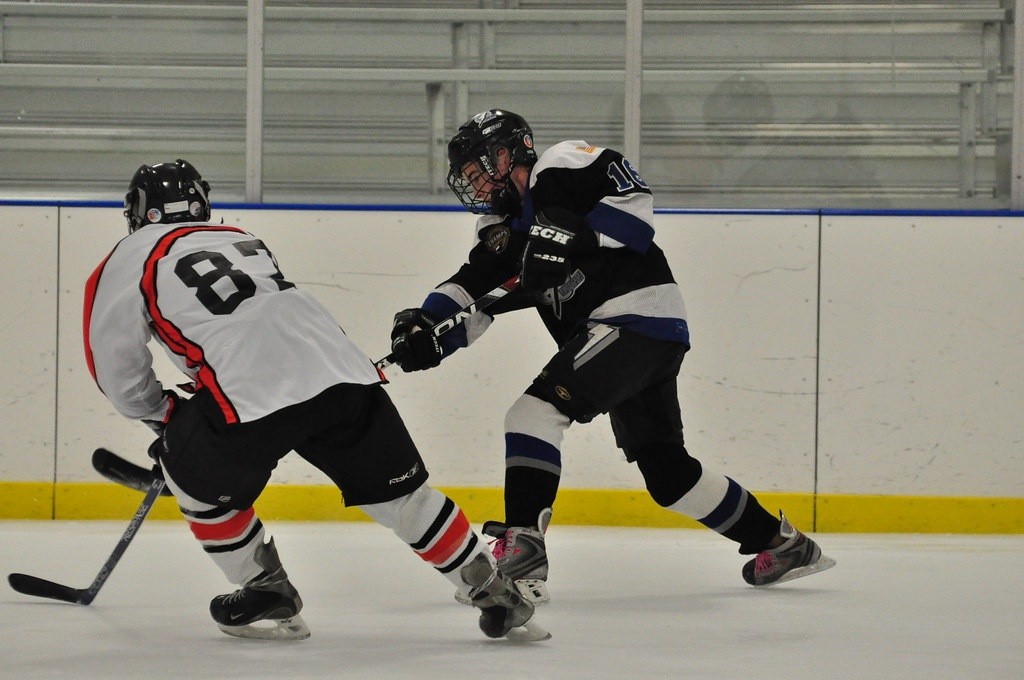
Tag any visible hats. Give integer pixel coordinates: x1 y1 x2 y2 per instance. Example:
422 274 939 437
390 308 443 373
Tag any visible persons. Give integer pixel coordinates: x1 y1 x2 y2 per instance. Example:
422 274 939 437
82 154 536 639
600 69 884 212
388 106 825 587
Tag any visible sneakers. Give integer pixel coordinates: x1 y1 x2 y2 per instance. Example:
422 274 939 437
209 535 311 640
742 508 836 589
455 508 554 604
455 557 551 646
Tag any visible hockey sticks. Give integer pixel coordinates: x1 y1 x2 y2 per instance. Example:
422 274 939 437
89 275 522 499
6 463 166 608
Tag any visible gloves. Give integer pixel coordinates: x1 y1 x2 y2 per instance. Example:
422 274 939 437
520 233 572 292
140 389 189 437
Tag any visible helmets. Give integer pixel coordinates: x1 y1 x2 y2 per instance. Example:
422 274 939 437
123 160 211 226
449 108 538 165
